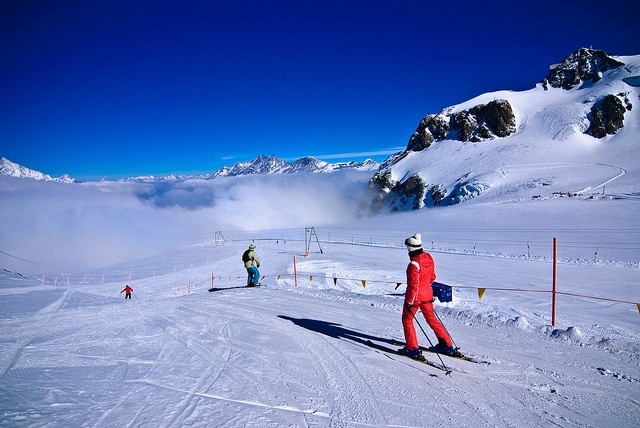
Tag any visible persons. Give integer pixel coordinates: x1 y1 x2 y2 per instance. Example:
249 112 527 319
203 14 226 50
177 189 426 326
242 243 261 287
121 285 134 298
402 233 453 356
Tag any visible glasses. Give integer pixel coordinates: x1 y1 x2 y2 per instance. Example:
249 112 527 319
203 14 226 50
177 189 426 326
405 239 421 247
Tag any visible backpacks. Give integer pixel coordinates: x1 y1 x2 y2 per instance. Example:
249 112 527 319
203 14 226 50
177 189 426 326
242 250 252 262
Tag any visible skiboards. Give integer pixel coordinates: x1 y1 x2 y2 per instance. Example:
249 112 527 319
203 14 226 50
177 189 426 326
367 339 490 371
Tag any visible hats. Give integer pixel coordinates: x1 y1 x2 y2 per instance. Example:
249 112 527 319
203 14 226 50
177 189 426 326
407 233 422 251
249 243 256 248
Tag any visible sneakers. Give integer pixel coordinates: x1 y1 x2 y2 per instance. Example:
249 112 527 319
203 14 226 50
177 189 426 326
253 283 259 286
435 343 453 353
402 347 420 356
248 284 253 287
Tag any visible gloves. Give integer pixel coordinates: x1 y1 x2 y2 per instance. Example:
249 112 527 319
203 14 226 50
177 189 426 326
257 265 259 268
405 303 413 312
